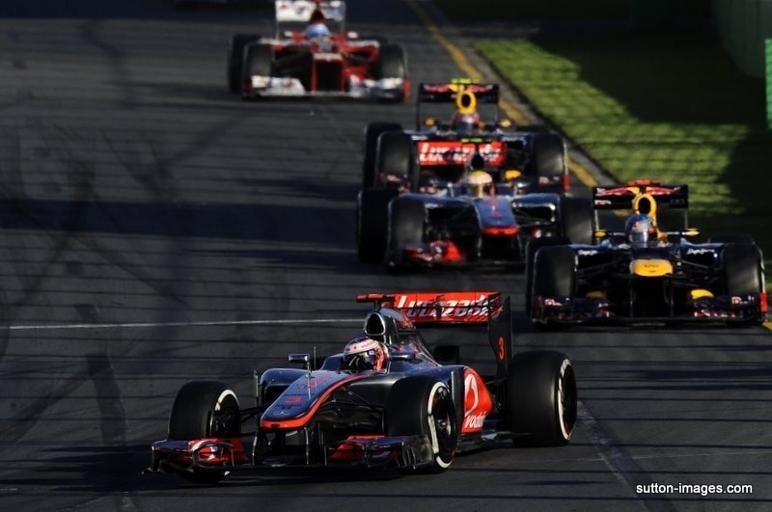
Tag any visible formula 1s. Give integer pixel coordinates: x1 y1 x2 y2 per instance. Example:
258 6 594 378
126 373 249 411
142 0 769 483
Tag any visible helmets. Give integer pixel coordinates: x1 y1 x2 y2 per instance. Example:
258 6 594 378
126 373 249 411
344 336 384 370
305 23 330 42
450 110 480 129
625 214 656 244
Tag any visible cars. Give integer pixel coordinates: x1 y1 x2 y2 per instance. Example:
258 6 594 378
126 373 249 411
142 0 769 483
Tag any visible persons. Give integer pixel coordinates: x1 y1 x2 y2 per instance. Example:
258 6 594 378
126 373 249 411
302 22 333 44
341 336 393 377
617 214 667 248
450 107 482 133
